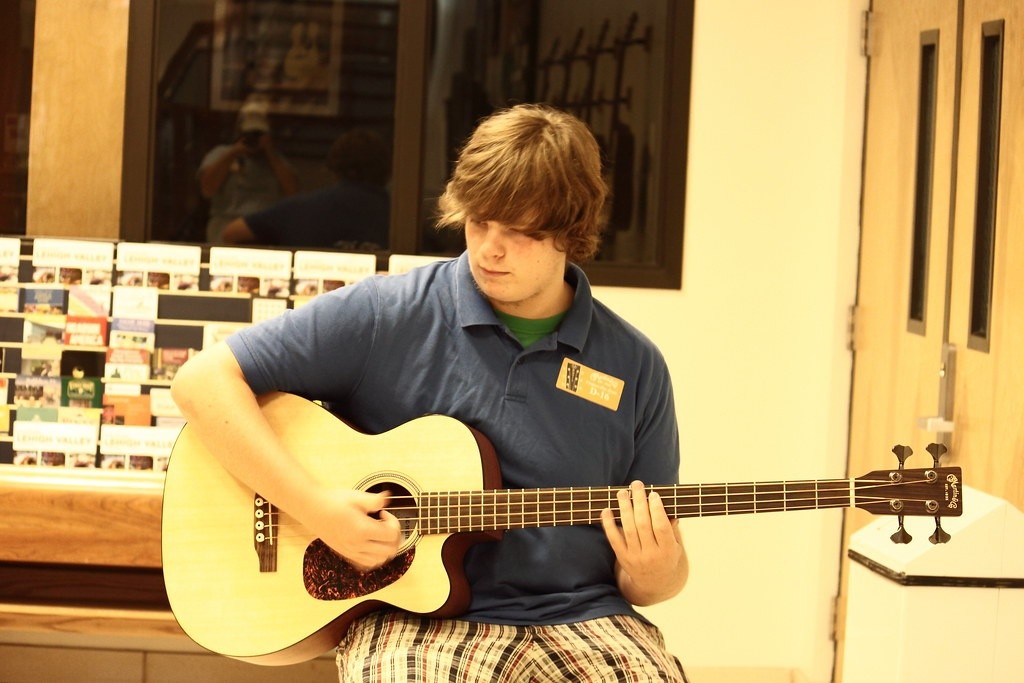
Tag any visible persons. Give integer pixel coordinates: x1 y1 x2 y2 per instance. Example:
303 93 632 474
194 104 302 244
222 127 390 252
169 104 689 683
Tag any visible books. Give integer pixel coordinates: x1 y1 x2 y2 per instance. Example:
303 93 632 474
0 237 457 472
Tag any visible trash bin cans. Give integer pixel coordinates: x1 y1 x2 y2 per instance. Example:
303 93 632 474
842 485 1024 683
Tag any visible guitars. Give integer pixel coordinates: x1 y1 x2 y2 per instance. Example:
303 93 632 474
159 386 963 666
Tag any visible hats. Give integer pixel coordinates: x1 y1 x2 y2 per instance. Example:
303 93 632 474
239 103 271 132
